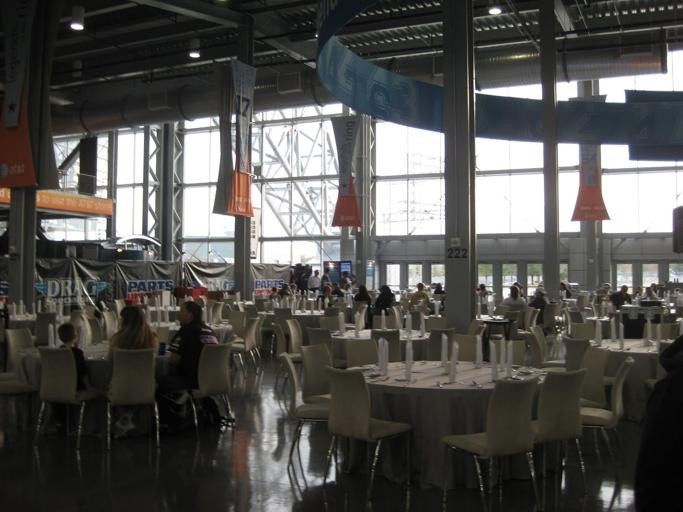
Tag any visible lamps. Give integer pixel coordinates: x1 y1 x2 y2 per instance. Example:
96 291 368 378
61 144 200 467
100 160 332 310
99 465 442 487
71 6 85 31
189 39 200 58
488 0 502 15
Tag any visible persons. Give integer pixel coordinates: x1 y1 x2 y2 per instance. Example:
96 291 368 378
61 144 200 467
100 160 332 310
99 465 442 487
93 283 115 321
474 269 683 329
55 326 100 436
266 268 445 330
105 308 152 434
155 300 221 433
631 334 683 511
171 279 191 305
